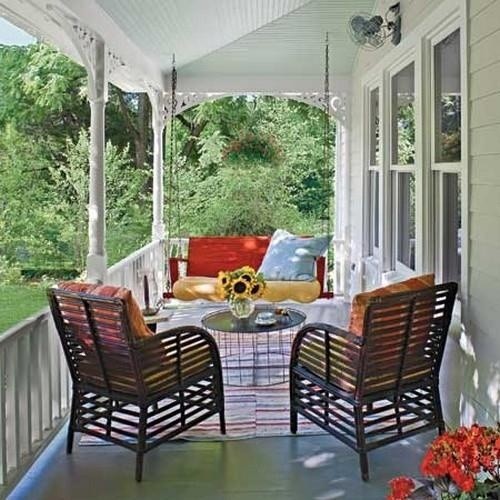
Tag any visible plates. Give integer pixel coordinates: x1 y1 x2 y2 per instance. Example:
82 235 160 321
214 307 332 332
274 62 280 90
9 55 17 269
255 319 276 325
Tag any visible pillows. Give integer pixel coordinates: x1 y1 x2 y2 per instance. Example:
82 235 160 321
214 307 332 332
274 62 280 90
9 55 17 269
57 281 166 376
344 272 435 389
256 228 335 281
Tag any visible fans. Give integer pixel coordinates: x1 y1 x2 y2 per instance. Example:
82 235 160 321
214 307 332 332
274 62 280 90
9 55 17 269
346 1 402 52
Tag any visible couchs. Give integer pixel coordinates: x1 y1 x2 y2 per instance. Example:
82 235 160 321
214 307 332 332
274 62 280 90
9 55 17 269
163 236 334 304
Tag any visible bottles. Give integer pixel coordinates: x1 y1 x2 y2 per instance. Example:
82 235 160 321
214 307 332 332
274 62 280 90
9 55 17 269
275 308 283 323
281 311 293 324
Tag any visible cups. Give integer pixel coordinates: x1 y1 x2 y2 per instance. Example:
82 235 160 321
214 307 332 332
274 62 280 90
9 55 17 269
257 312 272 322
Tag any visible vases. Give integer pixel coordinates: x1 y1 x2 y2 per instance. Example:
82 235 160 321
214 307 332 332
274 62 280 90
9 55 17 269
228 297 255 319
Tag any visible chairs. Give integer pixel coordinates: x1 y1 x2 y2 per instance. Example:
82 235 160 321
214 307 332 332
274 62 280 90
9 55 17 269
45 287 226 482
289 282 458 482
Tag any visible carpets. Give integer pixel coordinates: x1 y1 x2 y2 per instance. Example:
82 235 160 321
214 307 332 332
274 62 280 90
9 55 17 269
79 296 423 447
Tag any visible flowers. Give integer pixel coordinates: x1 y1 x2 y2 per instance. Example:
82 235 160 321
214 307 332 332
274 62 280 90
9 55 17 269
217 265 270 301
386 423 500 500
